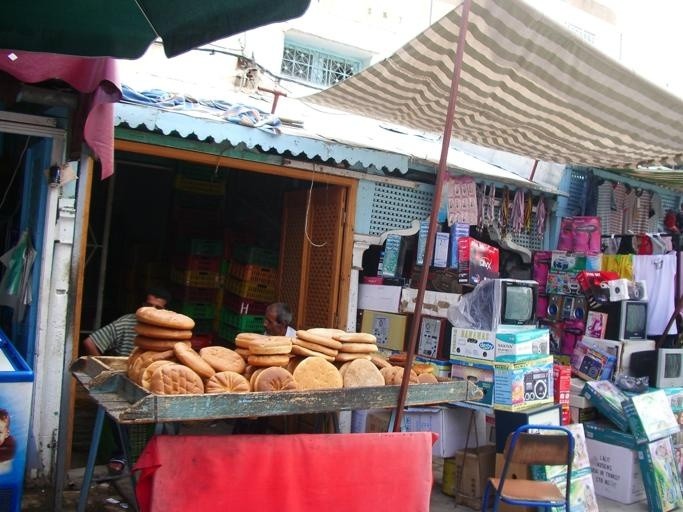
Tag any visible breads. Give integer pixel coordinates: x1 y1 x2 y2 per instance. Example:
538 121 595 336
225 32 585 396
128 306 438 396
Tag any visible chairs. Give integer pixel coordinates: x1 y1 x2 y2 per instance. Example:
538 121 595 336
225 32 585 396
483 425 576 512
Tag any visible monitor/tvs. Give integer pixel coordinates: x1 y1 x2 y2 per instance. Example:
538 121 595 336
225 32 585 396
464 278 539 333
592 300 649 341
629 348 683 388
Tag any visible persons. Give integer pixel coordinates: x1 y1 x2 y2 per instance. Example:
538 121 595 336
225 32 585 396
82 289 174 471
0 407 17 463
230 300 300 434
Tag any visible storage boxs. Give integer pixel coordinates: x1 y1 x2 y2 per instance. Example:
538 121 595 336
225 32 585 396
583 421 646 505
529 423 600 511
361 311 408 351
358 283 401 314
493 355 556 411
495 329 551 362
404 405 486 456
580 380 633 431
450 365 493 407
457 238 499 287
363 409 395 432
621 390 680 445
418 316 447 359
450 327 494 368
553 365 572 421
496 404 561 442
401 289 462 317
454 447 495 507
637 437 682 511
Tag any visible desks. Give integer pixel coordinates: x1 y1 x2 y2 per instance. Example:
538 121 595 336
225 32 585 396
68 355 483 512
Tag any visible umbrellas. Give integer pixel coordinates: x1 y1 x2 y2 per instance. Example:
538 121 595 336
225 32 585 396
0 1 315 60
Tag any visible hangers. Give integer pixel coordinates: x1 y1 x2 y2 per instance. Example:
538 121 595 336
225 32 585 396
636 181 644 194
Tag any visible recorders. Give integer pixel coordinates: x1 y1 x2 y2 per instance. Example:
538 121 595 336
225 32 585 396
553 255 576 271
563 277 582 295
524 371 549 400
579 356 602 380
546 294 587 323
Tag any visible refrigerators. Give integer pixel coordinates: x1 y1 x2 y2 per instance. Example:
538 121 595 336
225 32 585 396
0 327 34 512
